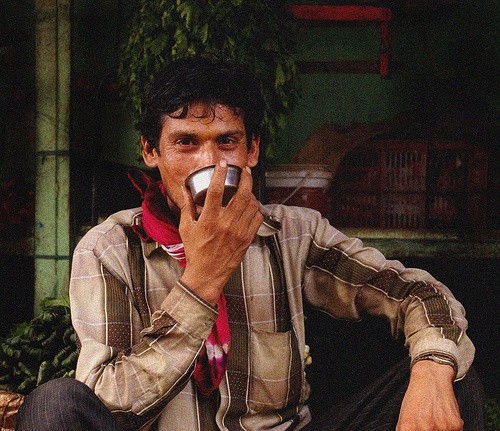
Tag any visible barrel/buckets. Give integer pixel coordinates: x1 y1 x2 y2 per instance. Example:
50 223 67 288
263 163 333 219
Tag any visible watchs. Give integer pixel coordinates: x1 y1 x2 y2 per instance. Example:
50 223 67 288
411 354 458 372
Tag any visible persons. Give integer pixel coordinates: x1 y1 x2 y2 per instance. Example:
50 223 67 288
14 52 488 431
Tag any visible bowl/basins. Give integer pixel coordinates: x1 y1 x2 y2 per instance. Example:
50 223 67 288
184 163 243 207
